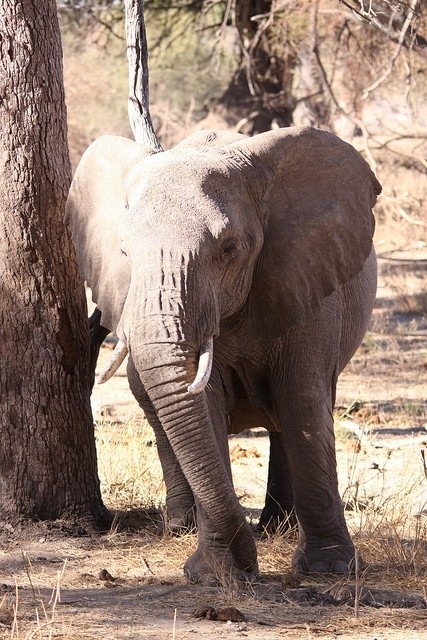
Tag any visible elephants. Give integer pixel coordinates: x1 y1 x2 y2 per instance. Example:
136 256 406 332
62 124 382 590
